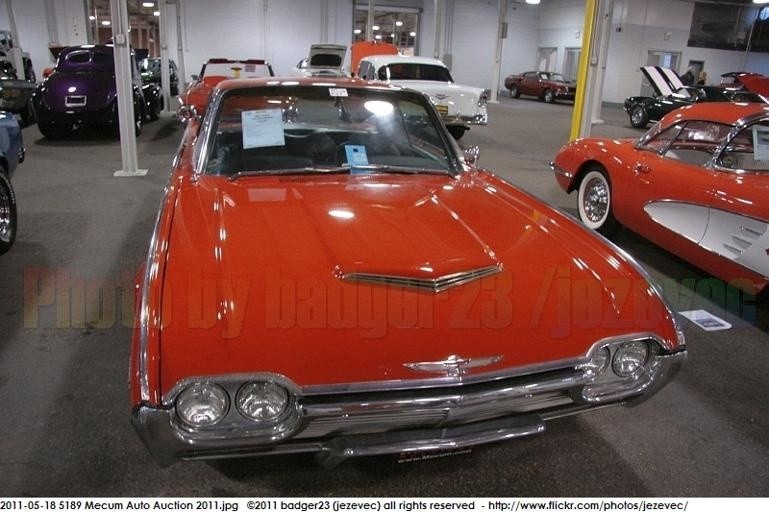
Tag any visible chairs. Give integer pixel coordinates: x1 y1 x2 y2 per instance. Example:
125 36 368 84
663 150 712 163
218 131 402 161
732 152 769 169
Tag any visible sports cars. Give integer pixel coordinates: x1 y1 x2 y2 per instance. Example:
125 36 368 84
0 35 769 467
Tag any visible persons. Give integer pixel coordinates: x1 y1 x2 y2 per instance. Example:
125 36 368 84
698 72 707 85
679 66 695 85
